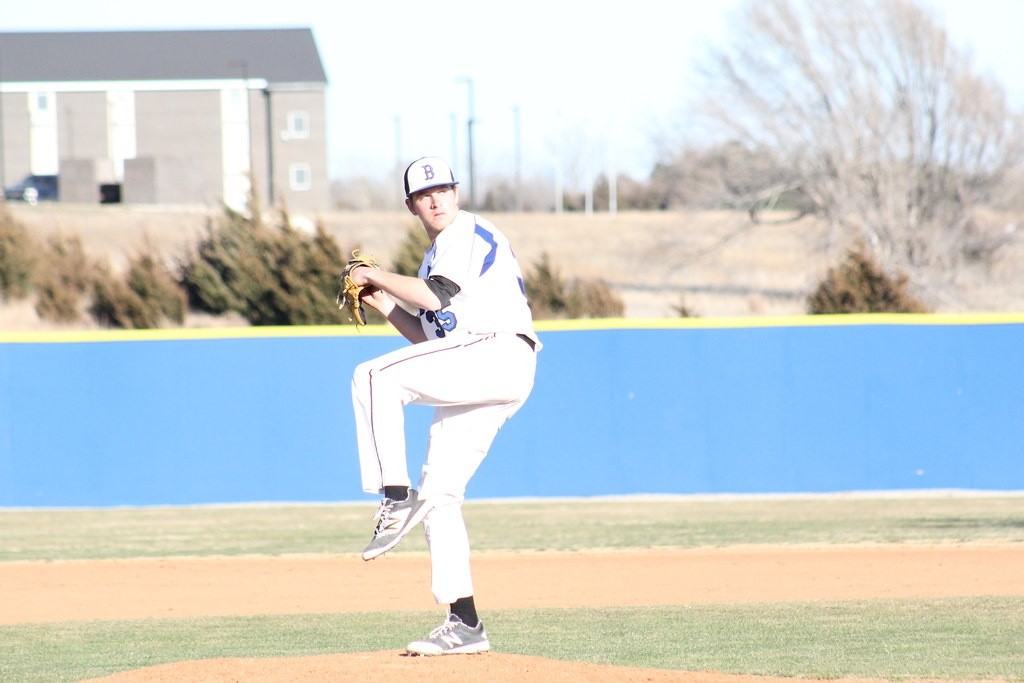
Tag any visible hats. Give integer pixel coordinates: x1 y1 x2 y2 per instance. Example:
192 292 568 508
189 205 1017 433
403 157 458 196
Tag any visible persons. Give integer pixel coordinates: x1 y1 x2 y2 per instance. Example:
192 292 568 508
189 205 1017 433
336 158 541 656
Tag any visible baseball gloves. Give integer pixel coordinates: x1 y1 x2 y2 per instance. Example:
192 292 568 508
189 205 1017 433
334 248 383 334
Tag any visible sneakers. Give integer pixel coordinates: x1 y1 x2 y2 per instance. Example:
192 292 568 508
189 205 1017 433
406 609 490 655
363 486 433 561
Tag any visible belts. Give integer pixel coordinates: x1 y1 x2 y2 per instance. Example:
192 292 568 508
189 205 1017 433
516 333 536 352
